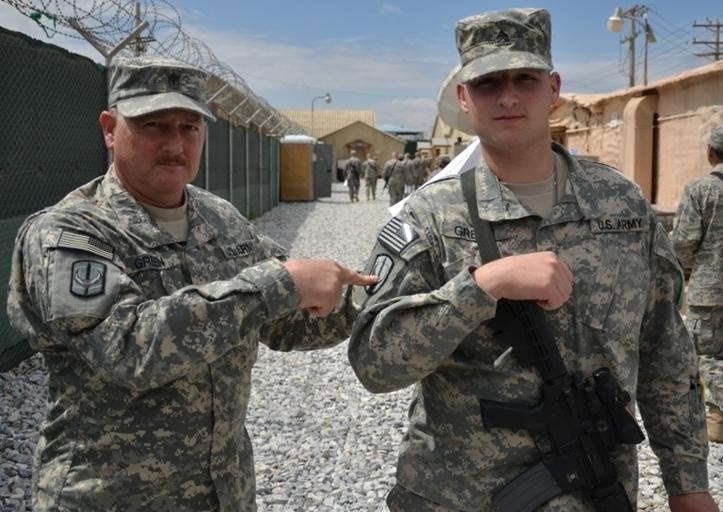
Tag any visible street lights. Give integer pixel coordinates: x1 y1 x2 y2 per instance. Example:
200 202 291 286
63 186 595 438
604 5 651 88
309 92 332 138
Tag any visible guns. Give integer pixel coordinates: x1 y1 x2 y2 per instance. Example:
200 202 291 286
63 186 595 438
477 298 645 511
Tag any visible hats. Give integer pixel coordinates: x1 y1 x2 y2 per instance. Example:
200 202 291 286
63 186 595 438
107 56 218 122
706 124 723 152
455 9 550 83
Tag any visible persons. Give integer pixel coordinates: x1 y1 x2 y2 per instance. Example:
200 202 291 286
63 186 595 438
381 146 456 206
341 149 363 204
0 53 385 511
362 152 375 202
663 125 722 444
338 5 718 512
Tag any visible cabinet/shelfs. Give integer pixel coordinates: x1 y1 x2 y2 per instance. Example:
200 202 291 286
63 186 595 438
279 140 334 203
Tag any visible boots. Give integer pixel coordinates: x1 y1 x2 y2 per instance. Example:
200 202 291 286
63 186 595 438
706 406 723 442
